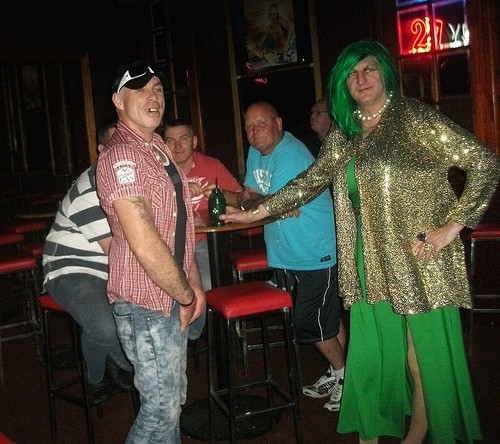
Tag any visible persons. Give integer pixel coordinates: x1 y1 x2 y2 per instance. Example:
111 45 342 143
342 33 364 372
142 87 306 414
41 39 498 444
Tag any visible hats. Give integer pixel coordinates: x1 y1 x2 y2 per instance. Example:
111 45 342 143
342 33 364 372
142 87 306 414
112 61 169 90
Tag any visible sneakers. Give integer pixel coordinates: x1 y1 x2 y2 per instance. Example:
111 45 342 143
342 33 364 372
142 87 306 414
303 364 344 411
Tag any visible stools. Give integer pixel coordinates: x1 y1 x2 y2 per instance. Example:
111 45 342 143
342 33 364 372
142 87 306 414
203 281 302 444
0 190 60 384
38 291 141 444
229 247 300 385
465 218 500 368
235 228 264 249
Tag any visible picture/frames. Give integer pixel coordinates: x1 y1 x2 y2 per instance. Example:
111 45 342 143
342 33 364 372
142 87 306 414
229 0 313 76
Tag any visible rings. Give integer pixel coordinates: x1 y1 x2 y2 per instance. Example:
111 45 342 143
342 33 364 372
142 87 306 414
421 240 434 254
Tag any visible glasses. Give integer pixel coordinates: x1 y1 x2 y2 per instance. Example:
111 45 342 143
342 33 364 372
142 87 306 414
308 111 329 116
117 65 161 93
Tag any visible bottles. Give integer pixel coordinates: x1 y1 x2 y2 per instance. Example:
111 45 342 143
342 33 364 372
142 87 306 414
208 189 226 226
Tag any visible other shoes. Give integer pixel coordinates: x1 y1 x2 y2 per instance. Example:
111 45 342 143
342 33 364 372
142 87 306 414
105 357 133 389
87 378 112 403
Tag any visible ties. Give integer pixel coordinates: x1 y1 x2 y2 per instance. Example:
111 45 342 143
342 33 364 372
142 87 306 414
152 144 187 269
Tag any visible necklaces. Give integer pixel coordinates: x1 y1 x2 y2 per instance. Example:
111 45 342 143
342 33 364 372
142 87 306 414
349 90 395 122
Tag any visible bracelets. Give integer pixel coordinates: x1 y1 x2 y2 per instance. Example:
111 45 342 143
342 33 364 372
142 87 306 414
180 291 194 306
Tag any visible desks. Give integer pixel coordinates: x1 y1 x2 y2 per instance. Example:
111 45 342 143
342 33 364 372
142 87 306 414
11 210 57 221
177 208 280 441
